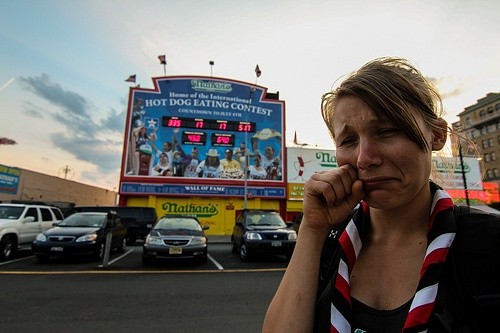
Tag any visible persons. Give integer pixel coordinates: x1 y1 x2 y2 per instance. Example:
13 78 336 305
259 56 500 332
125 127 282 181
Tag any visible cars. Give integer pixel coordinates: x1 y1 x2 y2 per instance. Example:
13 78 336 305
143 213 210 268
455 199 500 219
32 210 125 264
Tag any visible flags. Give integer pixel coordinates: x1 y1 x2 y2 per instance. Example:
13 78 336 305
158 55 166 63
256 65 261 77
125 74 135 82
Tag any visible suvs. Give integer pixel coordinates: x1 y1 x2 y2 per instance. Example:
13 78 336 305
231 208 297 263
0 204 66 261
